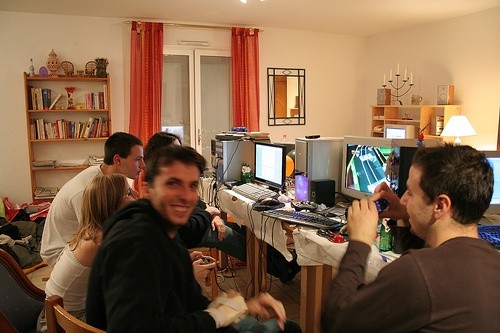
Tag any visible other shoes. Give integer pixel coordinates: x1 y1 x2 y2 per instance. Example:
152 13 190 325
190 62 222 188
280 250 301 283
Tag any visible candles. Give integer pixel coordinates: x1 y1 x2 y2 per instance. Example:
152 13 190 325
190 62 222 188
410 72 413 83
383 74 386 84
389 69 393 81
404 68 408 80
397 63 399 74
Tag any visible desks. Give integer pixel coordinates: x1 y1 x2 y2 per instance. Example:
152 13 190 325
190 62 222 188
77 70 84 76
208 182 401 333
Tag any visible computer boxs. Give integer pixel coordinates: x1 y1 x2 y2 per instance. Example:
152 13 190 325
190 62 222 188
216 139 271 185
295 137 343 203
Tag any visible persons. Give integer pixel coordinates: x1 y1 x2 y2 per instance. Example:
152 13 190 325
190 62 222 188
43 173 137 326
86 146 304 333
144 131 301 284
39 132 145 270
321 142 500 333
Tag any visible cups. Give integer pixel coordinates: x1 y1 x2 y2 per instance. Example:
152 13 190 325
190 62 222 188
435 115 443 136
412 95 422 104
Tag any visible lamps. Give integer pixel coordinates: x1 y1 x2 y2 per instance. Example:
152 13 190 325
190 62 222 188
441 115 477 144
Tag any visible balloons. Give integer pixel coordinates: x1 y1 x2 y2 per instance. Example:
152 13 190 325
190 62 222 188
285 149 296 179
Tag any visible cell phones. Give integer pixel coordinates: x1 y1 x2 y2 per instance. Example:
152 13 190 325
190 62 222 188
305 135 320 139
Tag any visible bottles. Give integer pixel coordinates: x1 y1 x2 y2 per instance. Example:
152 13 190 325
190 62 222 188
245 164 252 183
380 216 392 250
95 58 107 78
29 58 35 77
241 163 246 183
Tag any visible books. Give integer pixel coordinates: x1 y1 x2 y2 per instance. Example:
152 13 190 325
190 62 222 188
34 186 58 199
29 84 107 110
31 154 104 168
29 117 109 139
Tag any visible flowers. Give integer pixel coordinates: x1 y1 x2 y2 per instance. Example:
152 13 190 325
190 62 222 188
240 159 255 183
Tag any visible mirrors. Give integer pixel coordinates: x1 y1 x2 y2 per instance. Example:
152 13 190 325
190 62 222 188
267 67 306 125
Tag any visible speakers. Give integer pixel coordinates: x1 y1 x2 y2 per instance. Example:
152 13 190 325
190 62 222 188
311 178 335 208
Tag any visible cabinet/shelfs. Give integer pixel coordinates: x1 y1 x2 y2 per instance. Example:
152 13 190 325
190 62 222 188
371 104 461 144
22 70 112 201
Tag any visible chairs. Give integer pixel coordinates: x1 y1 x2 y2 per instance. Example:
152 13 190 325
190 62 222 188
61 61 75 76
0 248 46 333
85 61 98 76
45 295 107 333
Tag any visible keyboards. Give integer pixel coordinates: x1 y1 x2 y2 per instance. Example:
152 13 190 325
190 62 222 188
261 209 343 230
232 182 280 203
477 225 500 250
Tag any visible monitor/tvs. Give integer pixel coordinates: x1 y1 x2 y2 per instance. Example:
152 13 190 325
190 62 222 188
340 135 397 203
479 151 500 226
384 124 415 140
253 142 286 194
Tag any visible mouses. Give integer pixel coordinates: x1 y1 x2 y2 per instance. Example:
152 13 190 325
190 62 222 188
339 225 348 236
260 199 280 206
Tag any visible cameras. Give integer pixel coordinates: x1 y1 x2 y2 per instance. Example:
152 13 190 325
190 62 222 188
374 199 388 212
